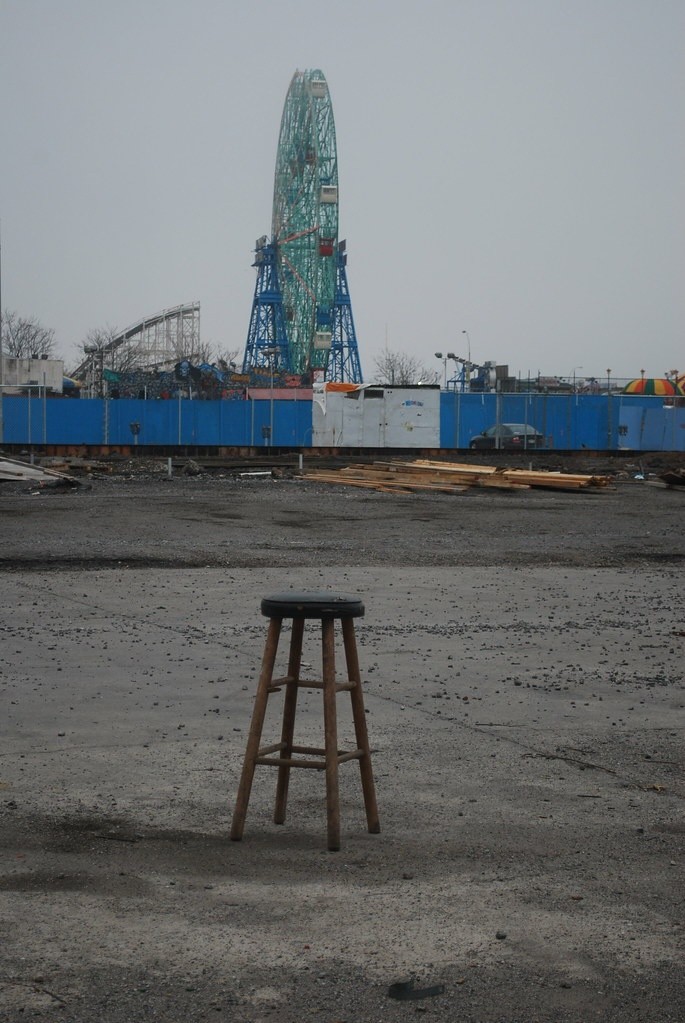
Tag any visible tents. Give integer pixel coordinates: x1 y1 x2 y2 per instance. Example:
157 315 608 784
620 374 685 406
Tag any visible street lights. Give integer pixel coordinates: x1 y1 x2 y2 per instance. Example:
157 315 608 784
84 346 97 399
435 352 455 387
640 368 645 395
568 367 582 384
260 348 276 446
462 331 470 392
606 368 612 395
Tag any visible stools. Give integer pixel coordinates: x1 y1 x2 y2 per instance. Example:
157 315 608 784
229 593 380 852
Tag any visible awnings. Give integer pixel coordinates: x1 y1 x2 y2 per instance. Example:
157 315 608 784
63 376 82 388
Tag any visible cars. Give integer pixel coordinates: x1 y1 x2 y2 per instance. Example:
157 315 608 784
470 424 543 449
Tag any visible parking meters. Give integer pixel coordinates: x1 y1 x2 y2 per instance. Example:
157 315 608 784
261 426 270 446
130 423 141 446
619 425 628 448
